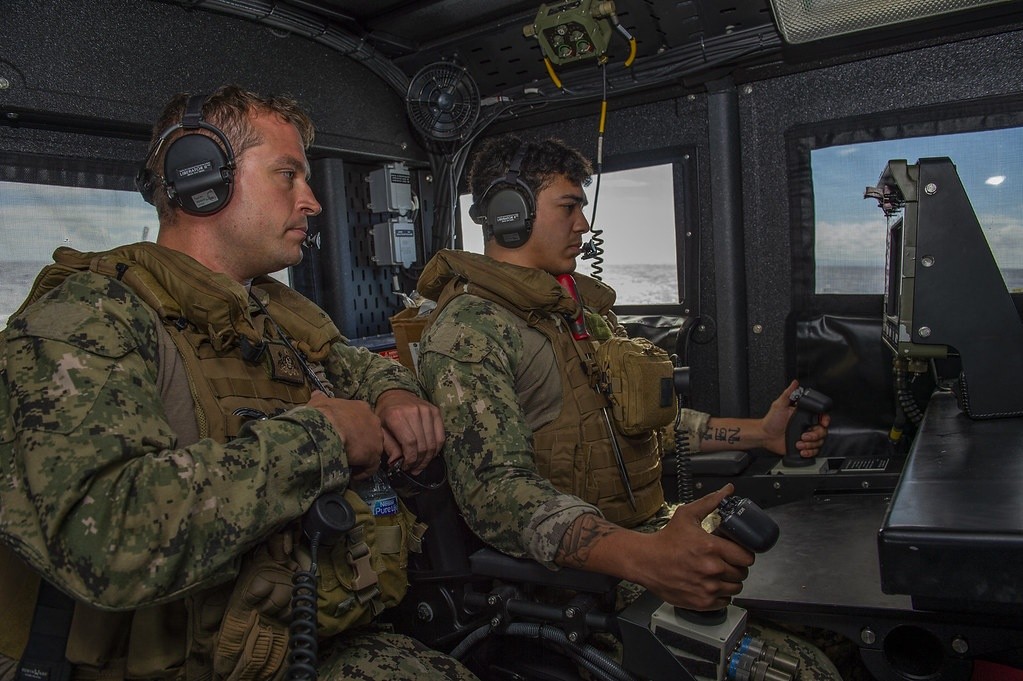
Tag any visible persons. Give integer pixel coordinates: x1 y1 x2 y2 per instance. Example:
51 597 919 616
417 136 849 681
1 87 482 680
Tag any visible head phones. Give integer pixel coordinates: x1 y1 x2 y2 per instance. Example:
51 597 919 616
467 142 536 248
135 95 235 214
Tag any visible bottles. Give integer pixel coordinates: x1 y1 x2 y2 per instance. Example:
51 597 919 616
351 470 400 516
